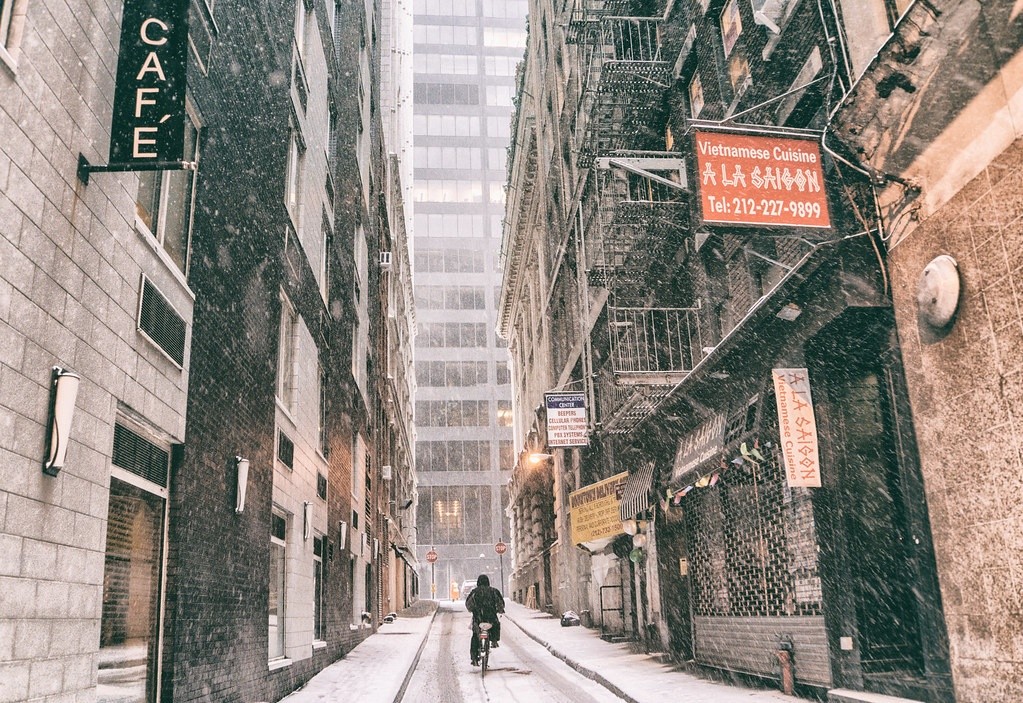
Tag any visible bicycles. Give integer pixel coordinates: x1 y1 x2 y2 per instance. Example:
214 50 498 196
468 608 506 677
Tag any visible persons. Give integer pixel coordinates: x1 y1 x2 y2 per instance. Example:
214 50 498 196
466 575 505 666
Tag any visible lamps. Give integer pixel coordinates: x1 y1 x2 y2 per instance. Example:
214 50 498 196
775 304 801 321
621 442 641 454
710 369 730 380
234 456 250 513
304 501 314 540
664 412 680 422
42 365 81 478
362 532 367 556
373 537 379 559
389 498 413 510
339 520 346 549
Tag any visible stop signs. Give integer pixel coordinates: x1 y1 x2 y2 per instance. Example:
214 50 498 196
426 552 438 562
495 543 506 554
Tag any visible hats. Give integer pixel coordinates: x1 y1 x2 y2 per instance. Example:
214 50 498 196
476 574 490 586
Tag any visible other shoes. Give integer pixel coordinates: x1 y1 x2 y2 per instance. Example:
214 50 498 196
491 642 499 648
471 659 478 666
